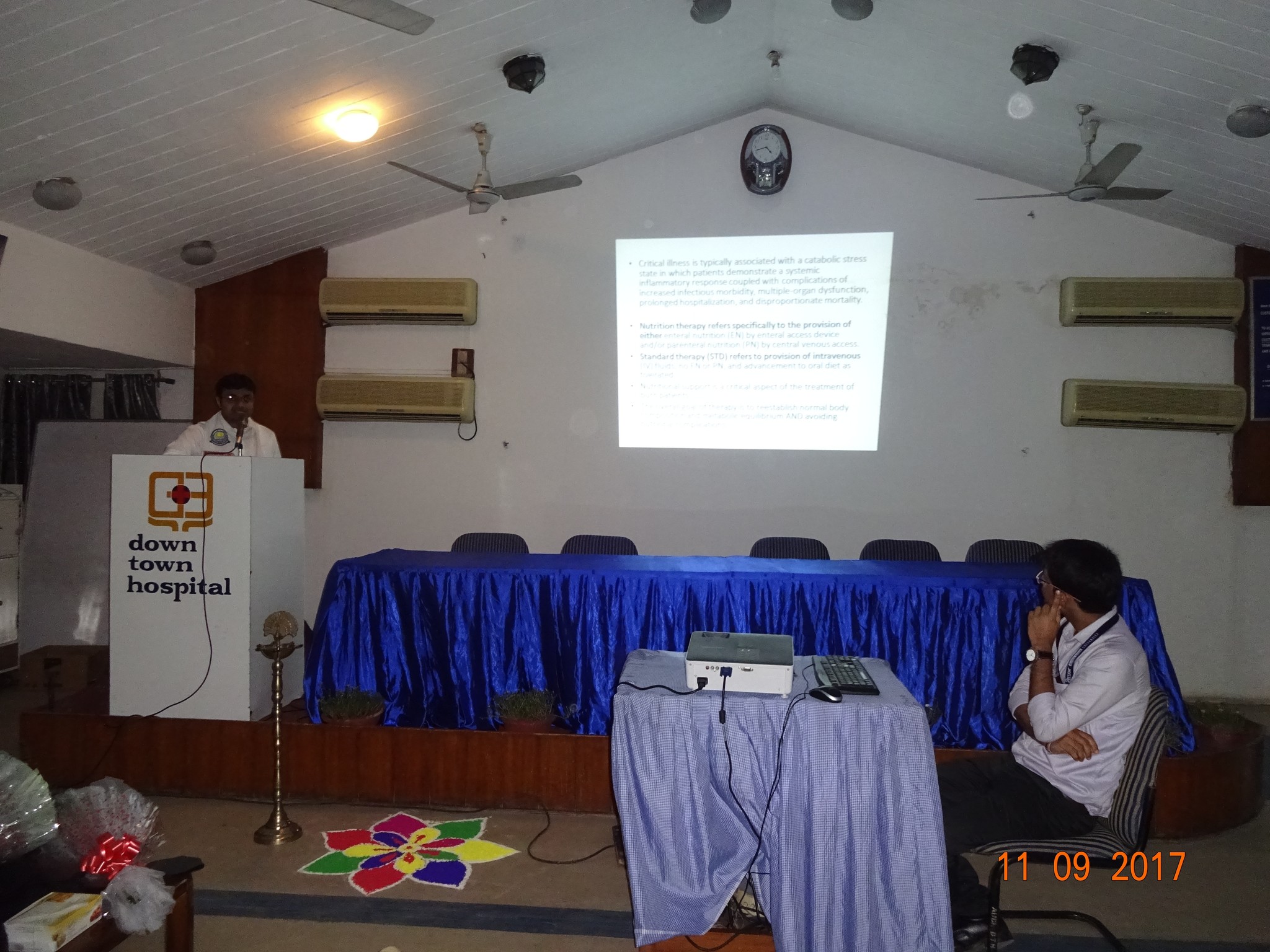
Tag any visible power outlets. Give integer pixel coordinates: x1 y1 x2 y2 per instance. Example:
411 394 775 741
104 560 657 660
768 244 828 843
451 348 475 379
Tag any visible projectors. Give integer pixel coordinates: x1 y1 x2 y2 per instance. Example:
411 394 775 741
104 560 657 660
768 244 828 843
685 630 794 698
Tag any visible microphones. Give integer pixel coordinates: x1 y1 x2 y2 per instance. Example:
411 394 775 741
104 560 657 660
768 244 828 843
235 415 248 446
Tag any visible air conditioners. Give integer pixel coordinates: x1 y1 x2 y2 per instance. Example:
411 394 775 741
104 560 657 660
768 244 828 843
315 372 474 424
1058 277 1246 330
318 276 479 325
1060 378 1247 436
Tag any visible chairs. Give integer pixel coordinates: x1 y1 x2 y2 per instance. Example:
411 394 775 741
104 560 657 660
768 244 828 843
858 539 943 561
749 537 831 561
965 681 1170 952
963 539 1045 565
449 532 530 554
560 534 639 556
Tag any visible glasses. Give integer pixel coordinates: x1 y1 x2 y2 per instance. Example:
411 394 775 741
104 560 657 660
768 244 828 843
1036 571 1082 607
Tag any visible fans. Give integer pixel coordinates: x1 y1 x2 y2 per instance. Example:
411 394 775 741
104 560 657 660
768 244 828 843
971 103 1173 204
386 122 582 216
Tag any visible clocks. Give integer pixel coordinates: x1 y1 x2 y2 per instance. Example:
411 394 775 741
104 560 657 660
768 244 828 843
740 124 793 197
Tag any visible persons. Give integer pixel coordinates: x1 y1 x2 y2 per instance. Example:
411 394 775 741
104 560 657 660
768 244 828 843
935 539 1155 951
161 372 281 459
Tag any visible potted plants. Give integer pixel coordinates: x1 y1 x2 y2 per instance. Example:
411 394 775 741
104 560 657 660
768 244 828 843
481 688 572 733
317 685 385 728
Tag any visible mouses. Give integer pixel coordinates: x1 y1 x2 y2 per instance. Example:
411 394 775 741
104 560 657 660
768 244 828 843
809 686 843 703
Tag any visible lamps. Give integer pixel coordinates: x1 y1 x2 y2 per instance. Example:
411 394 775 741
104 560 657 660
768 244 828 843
179 241 218 266
501 53 546 95
32 176 83 212
689 0 732 25
1225 104 1270 138
1009 42 1061 87
831 0 874 21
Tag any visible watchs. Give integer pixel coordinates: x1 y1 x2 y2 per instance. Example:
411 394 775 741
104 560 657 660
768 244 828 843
1024 648 1055 663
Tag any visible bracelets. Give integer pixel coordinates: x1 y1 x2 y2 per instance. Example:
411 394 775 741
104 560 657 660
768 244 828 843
1045 742 1053 753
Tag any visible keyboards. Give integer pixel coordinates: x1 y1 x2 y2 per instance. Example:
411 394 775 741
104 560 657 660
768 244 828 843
811 655 880 695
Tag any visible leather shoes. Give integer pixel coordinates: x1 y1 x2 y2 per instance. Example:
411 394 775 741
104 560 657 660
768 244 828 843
951 914 1014 952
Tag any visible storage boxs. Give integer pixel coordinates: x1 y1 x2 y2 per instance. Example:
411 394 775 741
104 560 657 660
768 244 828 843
3 891 104 952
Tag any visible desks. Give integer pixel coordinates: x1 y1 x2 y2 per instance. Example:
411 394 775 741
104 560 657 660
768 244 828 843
0 845 205 952
608 647 954 952
302 545 1196 761
